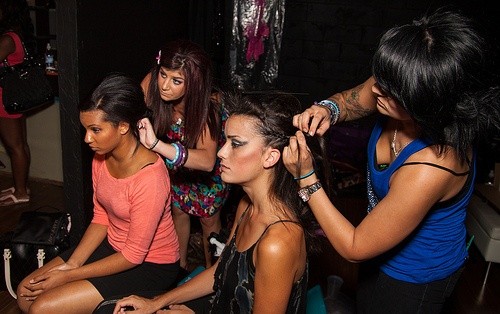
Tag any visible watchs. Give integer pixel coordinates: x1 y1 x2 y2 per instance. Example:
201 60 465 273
299 180 322 202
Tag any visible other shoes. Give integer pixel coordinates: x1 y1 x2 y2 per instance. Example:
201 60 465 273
0 187 32 206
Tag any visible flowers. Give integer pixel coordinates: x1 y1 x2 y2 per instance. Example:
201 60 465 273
155 50 161 65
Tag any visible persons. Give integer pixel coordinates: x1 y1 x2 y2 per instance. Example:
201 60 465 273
91 92 309 314
282 8 500 314
17 74 181 314
137 40 232 271
0 0 31 206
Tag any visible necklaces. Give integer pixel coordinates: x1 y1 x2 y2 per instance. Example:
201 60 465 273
391 127 417 158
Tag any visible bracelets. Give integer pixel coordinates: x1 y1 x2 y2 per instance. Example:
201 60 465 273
165 141 188 170
293 169 315 180
314 99 340 125
148 139 160 150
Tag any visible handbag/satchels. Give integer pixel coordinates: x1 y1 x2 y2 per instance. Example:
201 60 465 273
0 32 55 114
4 211 71 299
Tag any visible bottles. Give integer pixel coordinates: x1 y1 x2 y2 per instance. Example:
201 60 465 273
44 42 55 68
